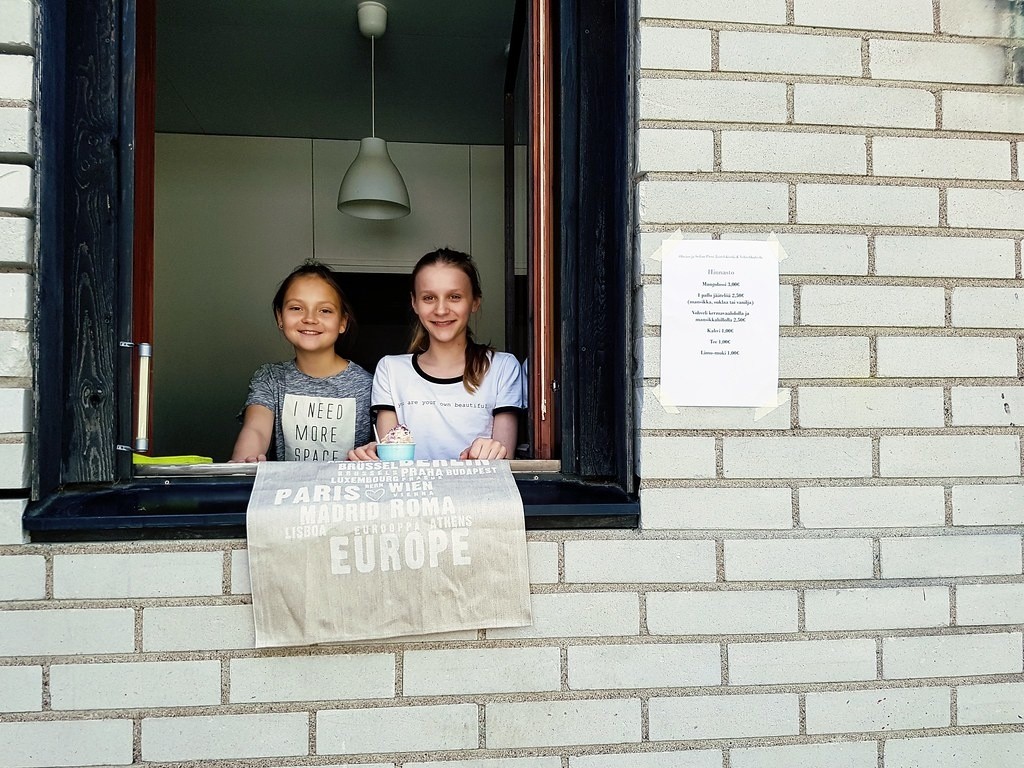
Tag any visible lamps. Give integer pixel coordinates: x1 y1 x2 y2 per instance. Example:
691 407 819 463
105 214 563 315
337 2 411 220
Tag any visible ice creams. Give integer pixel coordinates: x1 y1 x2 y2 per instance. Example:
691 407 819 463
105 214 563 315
376 423 414 444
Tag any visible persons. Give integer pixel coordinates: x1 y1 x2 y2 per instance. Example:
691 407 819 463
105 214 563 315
227 262 374 463
348 246 523 461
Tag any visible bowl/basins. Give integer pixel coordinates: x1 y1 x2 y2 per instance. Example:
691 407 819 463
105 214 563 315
376 443 416 461
